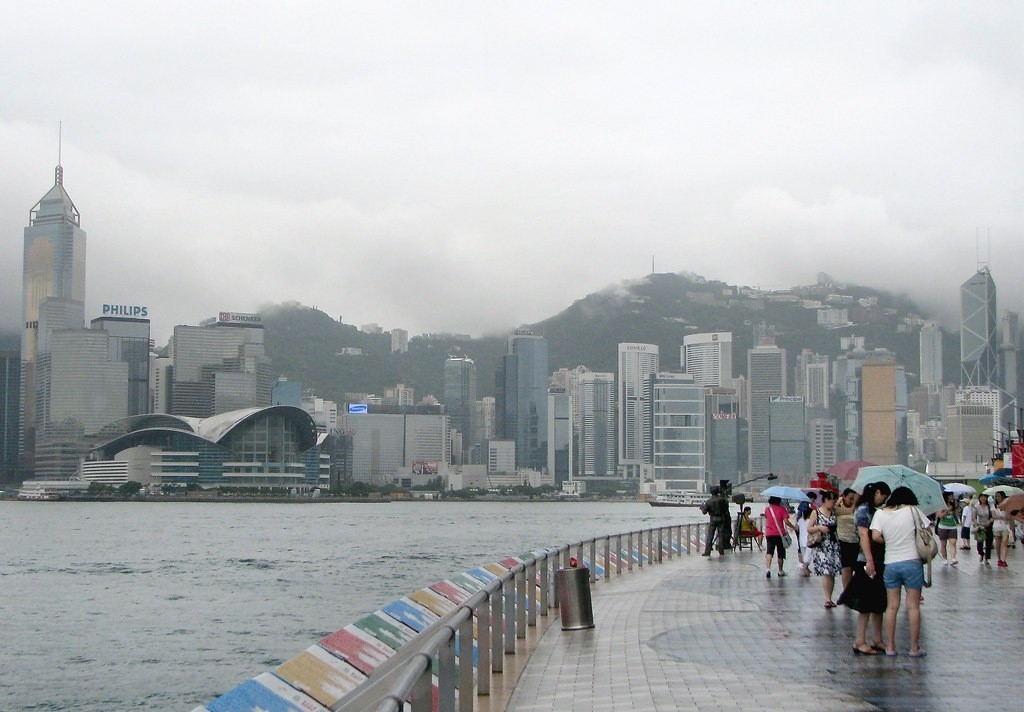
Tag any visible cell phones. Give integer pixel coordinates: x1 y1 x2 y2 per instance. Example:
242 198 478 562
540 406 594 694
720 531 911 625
864 566 877 579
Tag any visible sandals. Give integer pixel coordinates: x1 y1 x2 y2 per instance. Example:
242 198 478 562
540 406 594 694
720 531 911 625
871 638 888 651
824 601 837 608
853 641 878 656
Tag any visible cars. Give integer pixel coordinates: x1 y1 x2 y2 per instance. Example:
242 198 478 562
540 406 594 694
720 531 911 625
159 491 169 496
149 491 154 495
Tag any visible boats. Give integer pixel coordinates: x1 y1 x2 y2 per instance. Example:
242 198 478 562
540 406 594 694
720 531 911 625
648 491 721 507
781 498 795 514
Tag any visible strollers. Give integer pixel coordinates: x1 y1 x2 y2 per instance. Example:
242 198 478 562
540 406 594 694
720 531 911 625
795 524 813 576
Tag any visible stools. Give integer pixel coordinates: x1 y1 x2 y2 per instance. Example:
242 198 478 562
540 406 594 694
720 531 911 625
739 535 752 551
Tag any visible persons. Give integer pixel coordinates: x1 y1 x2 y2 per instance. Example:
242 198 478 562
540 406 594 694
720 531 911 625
741 507 764 548
998 493 1024 513
760 486 812 503
764 460 1024 657
700 486 727 556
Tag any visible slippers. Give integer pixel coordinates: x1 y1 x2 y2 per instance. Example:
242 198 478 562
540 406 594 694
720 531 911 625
909 644 927 657
885 647 897 656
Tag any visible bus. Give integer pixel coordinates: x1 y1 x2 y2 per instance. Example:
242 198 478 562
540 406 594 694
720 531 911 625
40 492 60 500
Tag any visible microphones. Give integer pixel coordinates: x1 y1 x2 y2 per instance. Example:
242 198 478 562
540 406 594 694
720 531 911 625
767 476 777 480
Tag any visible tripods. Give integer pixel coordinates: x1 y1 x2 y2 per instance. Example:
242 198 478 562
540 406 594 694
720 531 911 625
731 503 762 553
711 498 739 550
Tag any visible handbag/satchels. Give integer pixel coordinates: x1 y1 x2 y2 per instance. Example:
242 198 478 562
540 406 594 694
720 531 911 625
972 525 986 541
806 509 821 548
781 534 792 548
910 505 938 588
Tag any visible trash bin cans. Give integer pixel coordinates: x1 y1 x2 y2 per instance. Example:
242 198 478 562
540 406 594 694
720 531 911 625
555 567 596 631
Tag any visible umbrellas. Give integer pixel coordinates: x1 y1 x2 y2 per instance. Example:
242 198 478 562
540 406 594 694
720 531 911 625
978 474 1006 484
828 461 878 480
943 483 976 495
991 479 1023 488
849 464 949 519
982 484 1024 496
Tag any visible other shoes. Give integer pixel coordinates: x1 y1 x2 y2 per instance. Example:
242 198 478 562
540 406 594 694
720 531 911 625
814 572 822 576
757 546 766 550
766 570 771 578
1007 545 1011 547
978 553 985 562
943 559 948 564
778 571 785 576
984 560 989 565
803 570 810 577
702 552 710 556
719 550 724 555
997 560 1008 567
960 546 970 550
1012 545 1016 549
949 559 958 565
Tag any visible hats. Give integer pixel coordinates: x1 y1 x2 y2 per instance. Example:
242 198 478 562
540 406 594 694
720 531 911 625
959 498 969 503
